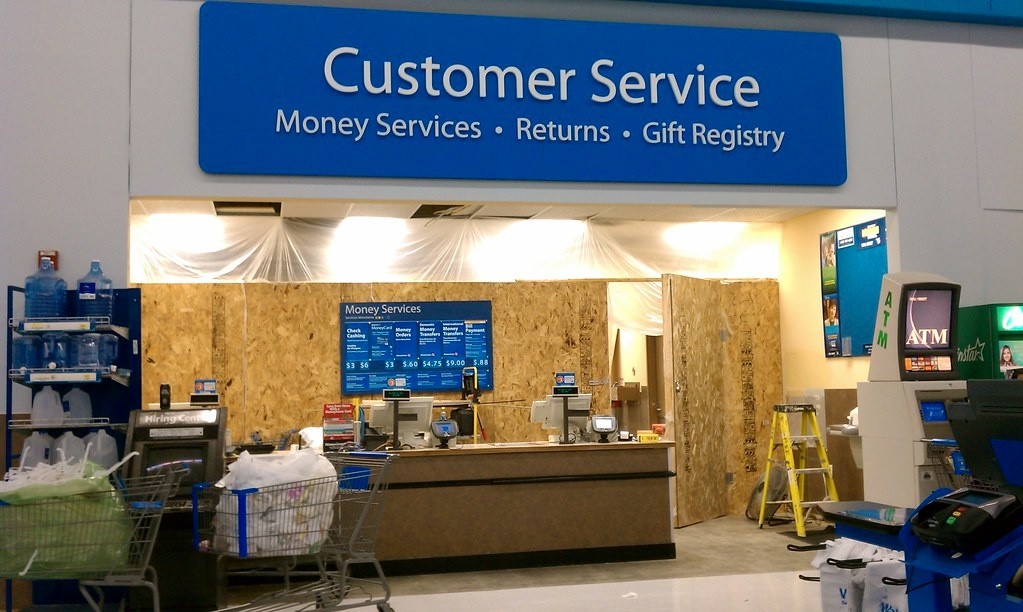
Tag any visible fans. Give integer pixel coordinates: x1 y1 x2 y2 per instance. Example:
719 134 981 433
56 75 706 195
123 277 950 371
745 466 792 527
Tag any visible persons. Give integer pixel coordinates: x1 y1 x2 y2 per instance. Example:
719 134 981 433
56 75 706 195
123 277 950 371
825 299 838 326
1000 346 1016 372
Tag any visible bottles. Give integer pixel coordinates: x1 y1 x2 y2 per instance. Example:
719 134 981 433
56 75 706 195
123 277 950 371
30 384 65 425
25 256 114 322
11 330 118 377
17 430 120 482
61 385 93 425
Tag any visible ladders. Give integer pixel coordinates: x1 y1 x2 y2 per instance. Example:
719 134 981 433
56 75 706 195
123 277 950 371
759 404 839 539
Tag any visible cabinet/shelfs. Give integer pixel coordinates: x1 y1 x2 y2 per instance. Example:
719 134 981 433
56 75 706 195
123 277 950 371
7 286 142 605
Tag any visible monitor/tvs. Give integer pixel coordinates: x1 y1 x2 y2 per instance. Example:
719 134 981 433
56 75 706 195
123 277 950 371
382 397 434 433
541 393 591 430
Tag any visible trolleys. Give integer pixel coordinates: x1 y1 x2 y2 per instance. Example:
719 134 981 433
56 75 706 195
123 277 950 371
0 458 194 612
191 448 398 612
